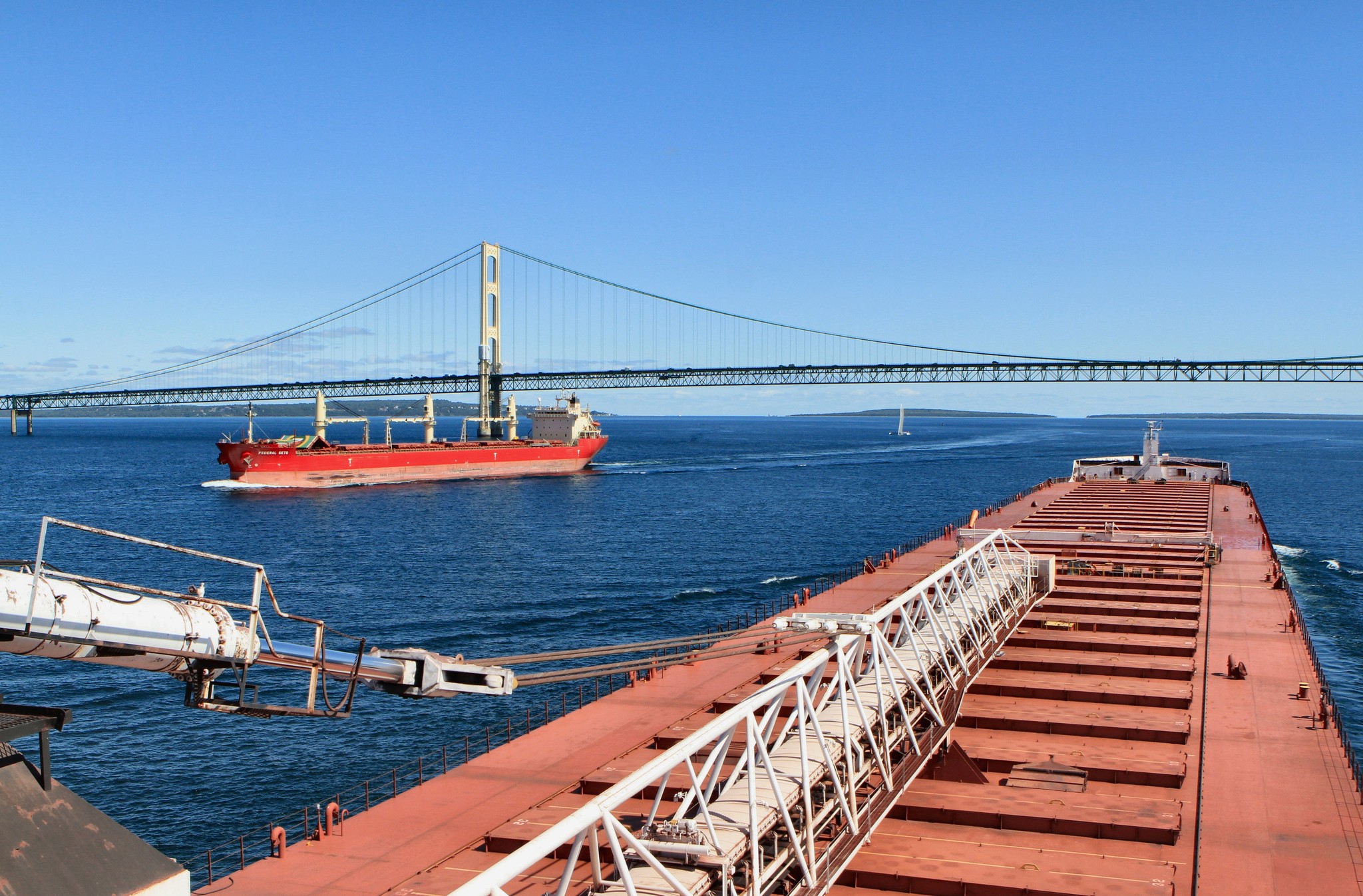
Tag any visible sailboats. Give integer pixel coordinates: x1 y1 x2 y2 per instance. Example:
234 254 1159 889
888 404 911 436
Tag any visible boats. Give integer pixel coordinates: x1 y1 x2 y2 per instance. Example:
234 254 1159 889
214 391 608 490
